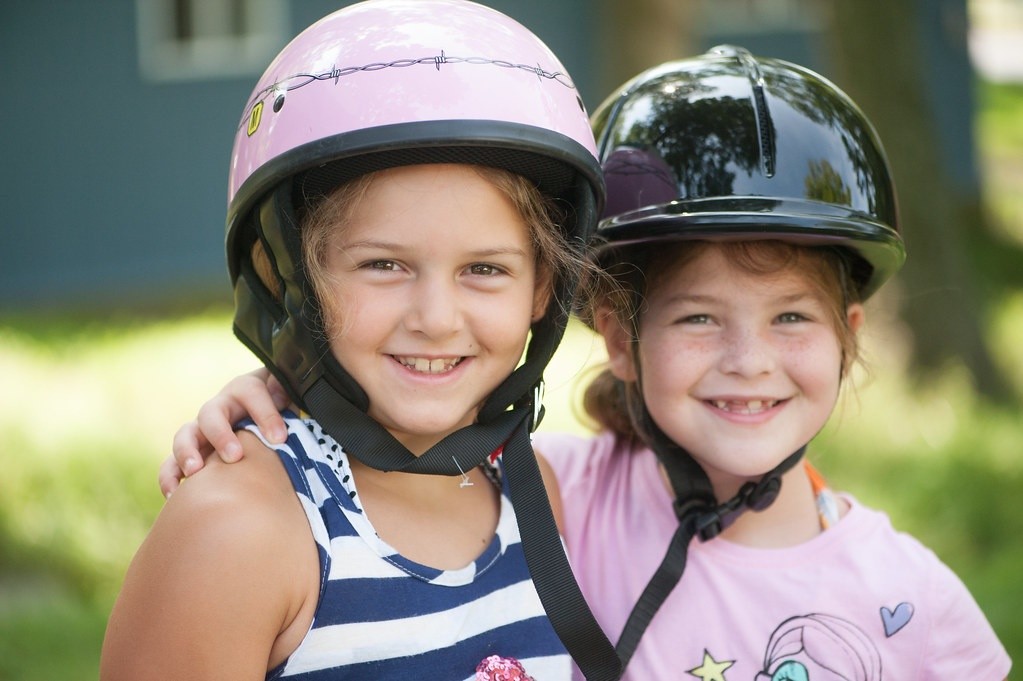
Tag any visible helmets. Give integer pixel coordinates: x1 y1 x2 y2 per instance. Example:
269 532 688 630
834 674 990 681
226 0 609 290
573 46 907 336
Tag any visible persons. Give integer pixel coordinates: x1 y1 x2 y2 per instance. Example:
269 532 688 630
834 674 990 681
99 0 605 681
158 44 1013 681
